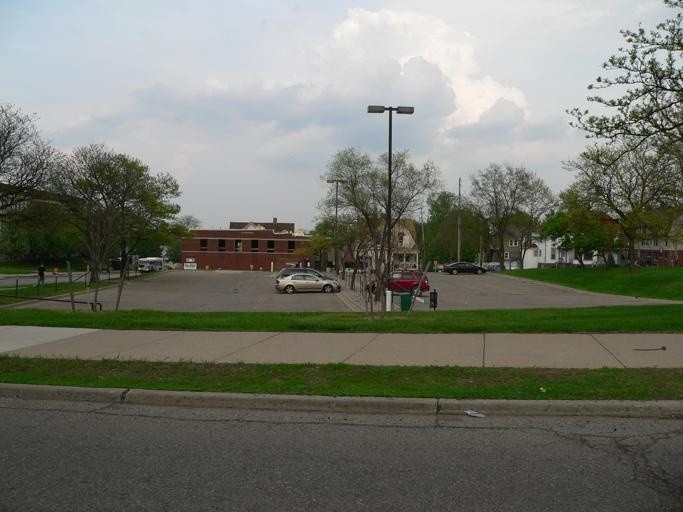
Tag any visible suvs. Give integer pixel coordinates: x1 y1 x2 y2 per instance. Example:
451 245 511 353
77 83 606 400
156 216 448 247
364 269 429 297
276 267 337 282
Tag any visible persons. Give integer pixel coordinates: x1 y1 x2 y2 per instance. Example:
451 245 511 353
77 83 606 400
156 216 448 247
37 263 45 285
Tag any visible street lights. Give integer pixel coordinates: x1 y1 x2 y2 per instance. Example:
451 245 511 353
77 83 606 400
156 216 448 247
326 178 349 276
365 104 415 312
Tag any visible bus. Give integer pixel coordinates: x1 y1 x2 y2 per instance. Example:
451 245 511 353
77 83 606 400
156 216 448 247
137 257 173 274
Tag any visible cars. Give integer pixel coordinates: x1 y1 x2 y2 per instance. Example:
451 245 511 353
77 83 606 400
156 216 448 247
442 261 488 275
275 272 337 293
111 256 131 269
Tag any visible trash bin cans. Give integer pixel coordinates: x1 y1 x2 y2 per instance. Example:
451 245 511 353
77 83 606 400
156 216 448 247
400 293 412 312
